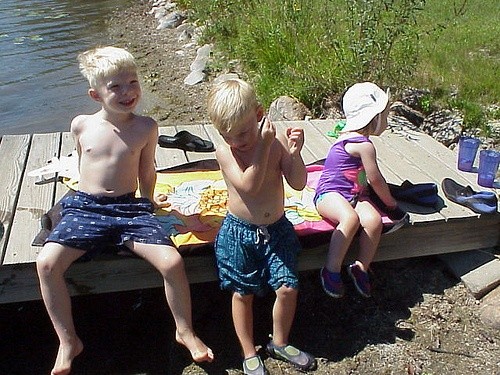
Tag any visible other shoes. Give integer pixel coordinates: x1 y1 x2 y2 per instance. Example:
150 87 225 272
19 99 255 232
321 265 346 298
348 261 373 297
442 177 498 216
243 340 316 375
387 180 443 207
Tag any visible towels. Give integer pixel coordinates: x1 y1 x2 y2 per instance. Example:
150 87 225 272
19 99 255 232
30 156 412 255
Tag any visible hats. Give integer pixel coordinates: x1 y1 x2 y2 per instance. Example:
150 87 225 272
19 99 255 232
340 81 387 133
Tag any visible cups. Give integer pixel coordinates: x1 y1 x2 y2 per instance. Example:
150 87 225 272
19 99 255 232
477 150 500 187
457 136 480 172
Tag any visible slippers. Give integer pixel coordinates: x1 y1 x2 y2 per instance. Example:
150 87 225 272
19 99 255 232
158 130 214 152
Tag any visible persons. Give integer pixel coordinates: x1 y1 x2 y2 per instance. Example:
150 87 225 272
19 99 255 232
208 79 315 375
38 45 214 375
313 81 407 299
